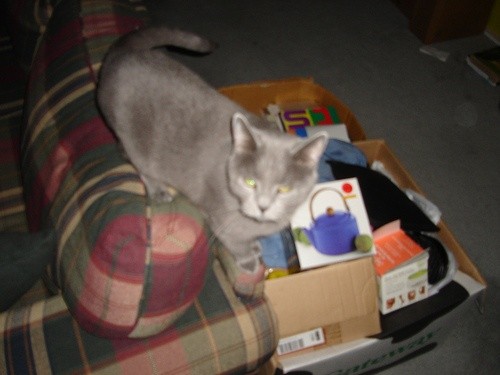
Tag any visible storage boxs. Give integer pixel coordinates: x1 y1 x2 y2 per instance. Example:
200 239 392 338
258 139 487 375
215 77 367 143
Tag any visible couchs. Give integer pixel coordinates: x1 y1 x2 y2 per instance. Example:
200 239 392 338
0 0 279 375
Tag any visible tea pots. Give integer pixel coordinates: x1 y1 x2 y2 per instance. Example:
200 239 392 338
303 187 360 254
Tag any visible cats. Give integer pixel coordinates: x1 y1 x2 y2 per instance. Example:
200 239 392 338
93 23 330 275
